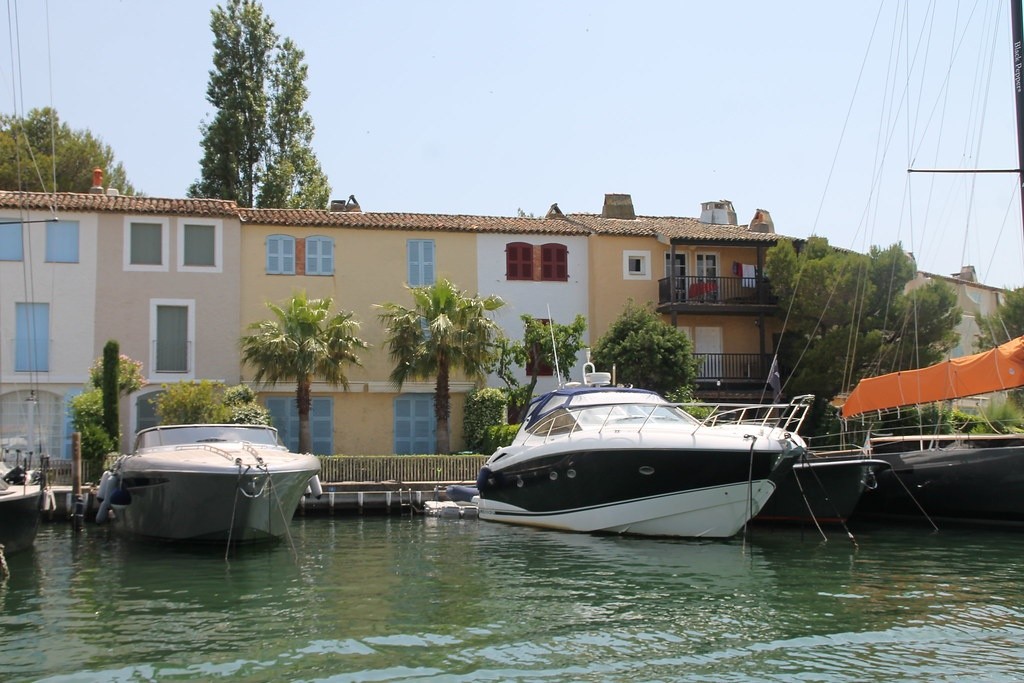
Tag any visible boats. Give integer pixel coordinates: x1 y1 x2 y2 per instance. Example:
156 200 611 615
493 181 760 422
447 303 814 542
93 422 322 556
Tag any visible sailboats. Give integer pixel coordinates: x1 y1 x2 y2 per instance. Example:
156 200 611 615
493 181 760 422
733 1 1024 532
0 0 59 551
